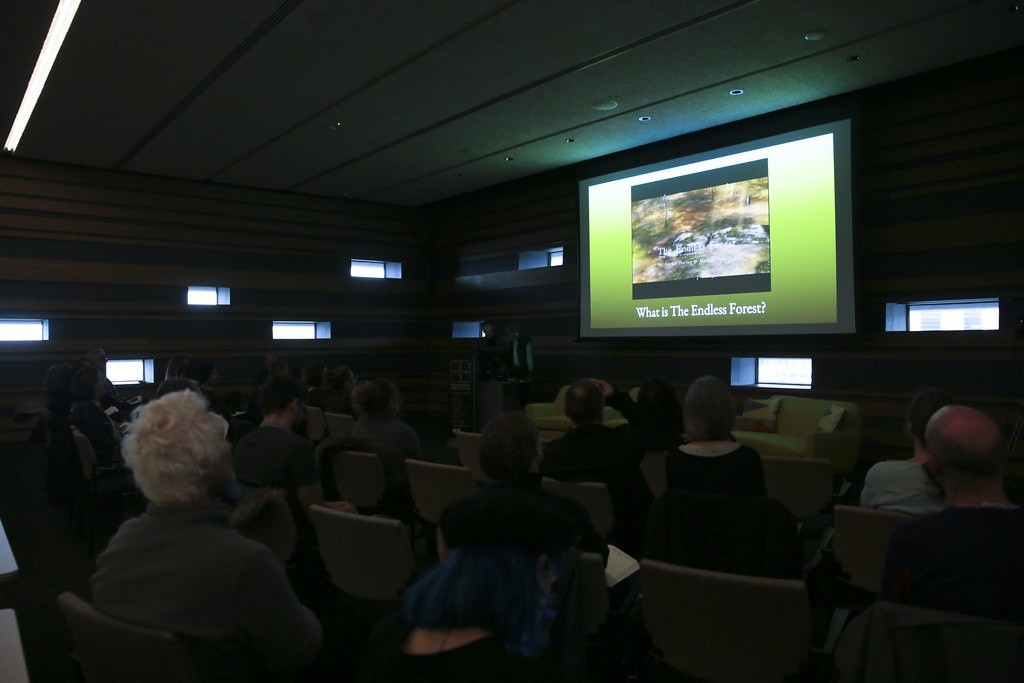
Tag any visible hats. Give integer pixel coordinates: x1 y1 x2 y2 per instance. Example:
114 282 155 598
188 360 214 386
439 485 580 557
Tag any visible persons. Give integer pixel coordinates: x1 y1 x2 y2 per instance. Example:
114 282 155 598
3 336 1024 680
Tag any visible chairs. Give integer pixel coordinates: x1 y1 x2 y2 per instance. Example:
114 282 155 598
2 380 1024 683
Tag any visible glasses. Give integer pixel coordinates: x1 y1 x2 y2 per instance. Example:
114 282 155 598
96 356 109 362
346 375 358 382
